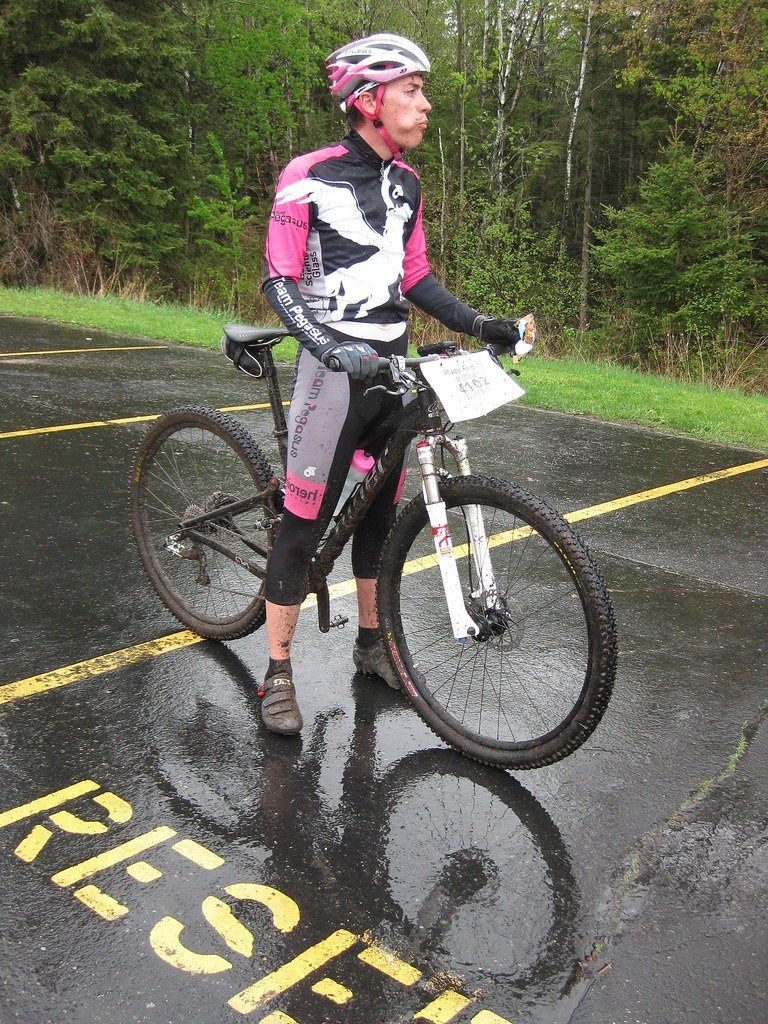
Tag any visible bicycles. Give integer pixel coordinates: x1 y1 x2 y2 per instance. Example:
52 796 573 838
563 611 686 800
132 626 578 994
128 323 620 771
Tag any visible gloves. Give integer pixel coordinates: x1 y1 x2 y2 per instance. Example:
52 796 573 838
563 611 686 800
321 340 379 384
480 316 521 351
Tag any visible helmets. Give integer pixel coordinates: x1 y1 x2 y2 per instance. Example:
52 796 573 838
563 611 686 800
324 33 431 112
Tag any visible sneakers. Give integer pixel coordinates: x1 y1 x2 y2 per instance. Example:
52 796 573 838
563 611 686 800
352 641 426 690
259 672 304 734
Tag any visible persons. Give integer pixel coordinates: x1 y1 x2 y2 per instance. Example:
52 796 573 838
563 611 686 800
258 33 536 735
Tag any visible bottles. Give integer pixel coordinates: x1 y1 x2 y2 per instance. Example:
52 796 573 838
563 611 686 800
332 449 375 517
